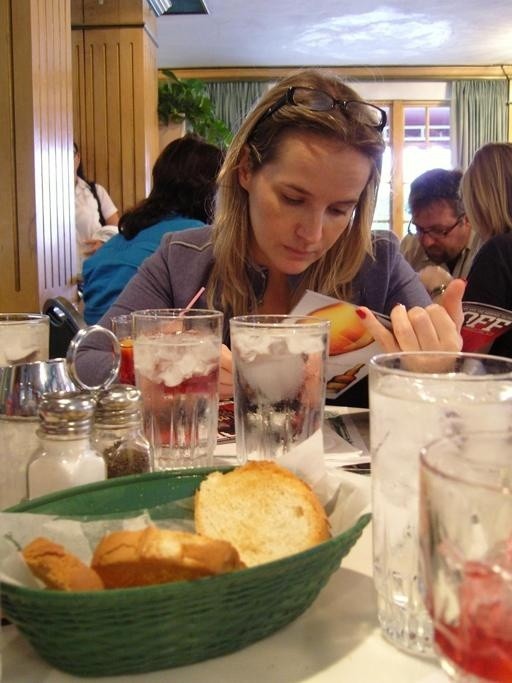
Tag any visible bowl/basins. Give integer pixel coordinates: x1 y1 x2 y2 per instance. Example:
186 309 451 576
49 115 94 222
0 459 373 680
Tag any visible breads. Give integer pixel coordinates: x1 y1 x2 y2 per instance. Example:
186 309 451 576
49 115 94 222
23 459 333 594
294 302 374 356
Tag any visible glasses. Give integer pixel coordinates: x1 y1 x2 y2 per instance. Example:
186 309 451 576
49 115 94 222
415 211 467 239
255 83 386 133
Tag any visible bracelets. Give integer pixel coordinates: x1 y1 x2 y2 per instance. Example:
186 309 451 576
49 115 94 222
428 284 448 295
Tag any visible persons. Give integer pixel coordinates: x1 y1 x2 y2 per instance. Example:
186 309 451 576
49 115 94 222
399 166 480 282
414 142 512 373
81 136 226 328
70 68 464 391
72 140 123 283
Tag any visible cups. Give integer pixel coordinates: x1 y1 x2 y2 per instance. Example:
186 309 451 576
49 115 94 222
361 347 511 663
0 310 51 372
111 307 332 468
410 425 510 681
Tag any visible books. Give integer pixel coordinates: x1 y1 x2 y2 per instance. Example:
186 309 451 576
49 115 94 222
280 286 511 400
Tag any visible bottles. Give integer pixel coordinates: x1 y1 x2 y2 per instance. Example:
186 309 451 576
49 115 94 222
26 385 155 505
